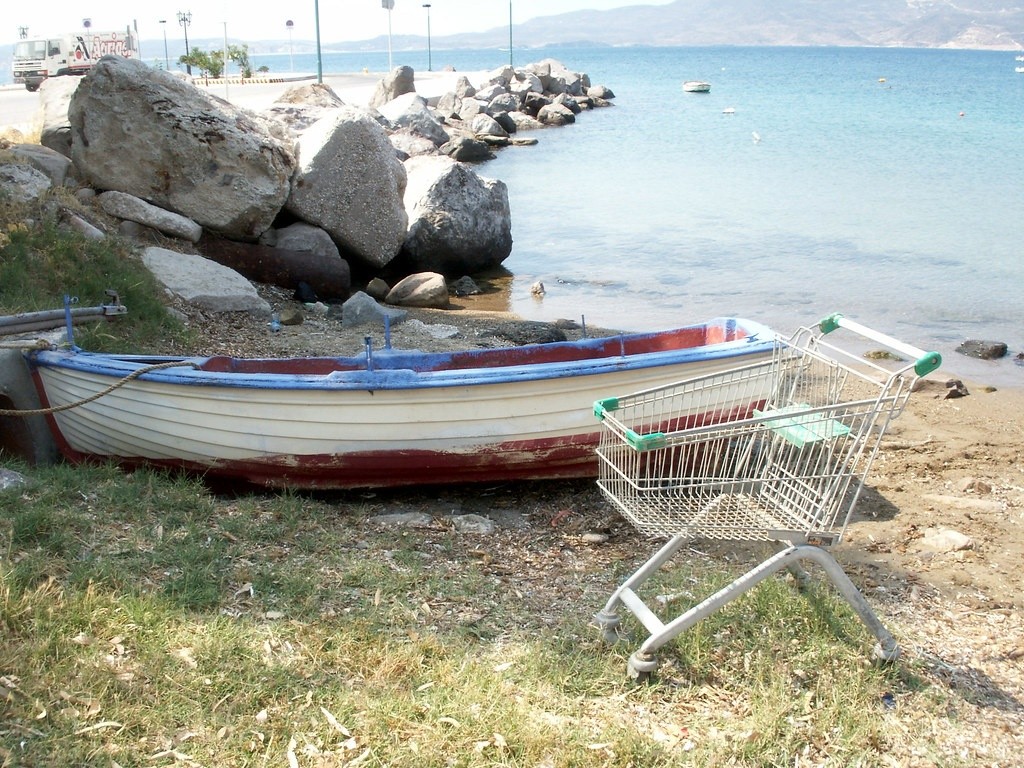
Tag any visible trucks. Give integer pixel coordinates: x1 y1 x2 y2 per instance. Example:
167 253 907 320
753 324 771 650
11 29 134 92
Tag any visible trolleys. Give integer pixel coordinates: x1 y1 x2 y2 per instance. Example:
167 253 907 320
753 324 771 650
590 314 944 679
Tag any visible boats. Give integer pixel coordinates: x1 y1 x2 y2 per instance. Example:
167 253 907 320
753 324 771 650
23 317 790 489
682 79 712 92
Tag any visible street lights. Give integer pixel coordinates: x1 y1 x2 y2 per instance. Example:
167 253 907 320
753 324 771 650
423 2 431 70
159 19 170 71
